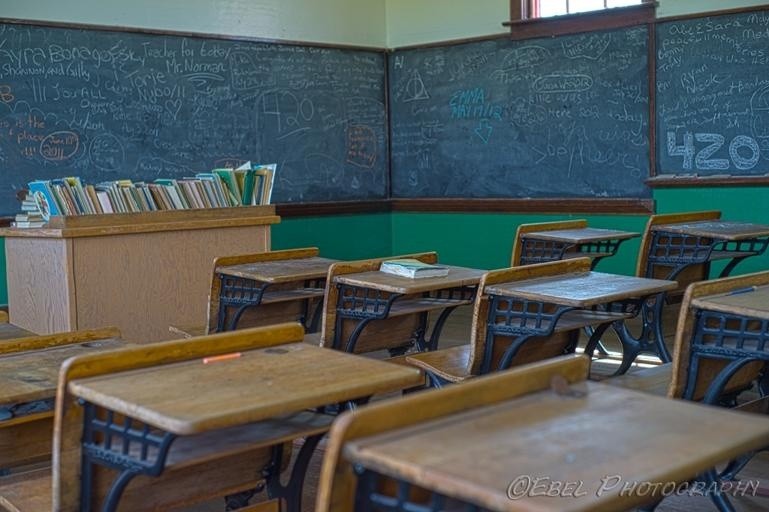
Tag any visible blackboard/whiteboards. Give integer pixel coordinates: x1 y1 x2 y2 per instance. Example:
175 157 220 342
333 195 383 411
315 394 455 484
388 18 656 215
0 18 391 227
653 5 769 188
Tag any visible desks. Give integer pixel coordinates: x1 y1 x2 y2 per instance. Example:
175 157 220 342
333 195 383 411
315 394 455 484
0 205 769 511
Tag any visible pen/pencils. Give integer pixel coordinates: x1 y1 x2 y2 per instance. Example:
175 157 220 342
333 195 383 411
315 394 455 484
203 351 246 365
726 286 757 296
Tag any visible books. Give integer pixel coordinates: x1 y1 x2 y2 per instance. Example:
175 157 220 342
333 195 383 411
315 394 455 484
10 160 277 228
380 258 449 279
